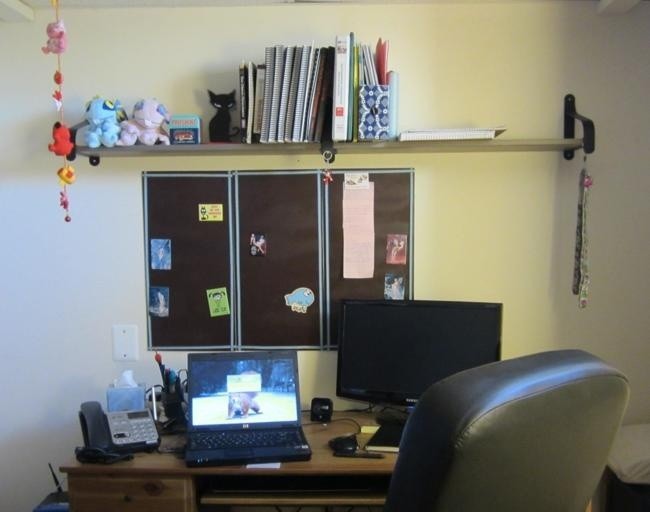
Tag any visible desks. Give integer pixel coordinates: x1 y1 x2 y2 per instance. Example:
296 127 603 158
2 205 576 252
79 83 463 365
60 404 593 512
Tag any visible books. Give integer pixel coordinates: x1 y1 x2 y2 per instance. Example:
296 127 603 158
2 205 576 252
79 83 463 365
364 424 404 452
402 127 506 137
235 29 401 146
398 130 508 142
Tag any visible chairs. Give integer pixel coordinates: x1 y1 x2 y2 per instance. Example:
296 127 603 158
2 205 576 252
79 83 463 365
386 350 630 509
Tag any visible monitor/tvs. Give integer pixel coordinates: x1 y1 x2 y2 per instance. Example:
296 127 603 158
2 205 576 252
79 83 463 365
336 299 502 413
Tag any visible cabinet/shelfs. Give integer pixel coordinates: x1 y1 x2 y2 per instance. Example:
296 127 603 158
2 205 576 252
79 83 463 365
64 93 597 176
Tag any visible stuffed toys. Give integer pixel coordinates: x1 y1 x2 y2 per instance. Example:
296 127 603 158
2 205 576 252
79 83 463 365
205 88 239 143
47 120 75 156
40 18 68 56
58 192 69 210
57 165 76 185
75 93 128 147
116 96 172 146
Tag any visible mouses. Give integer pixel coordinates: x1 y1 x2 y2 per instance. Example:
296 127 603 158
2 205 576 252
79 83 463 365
328 435 357 453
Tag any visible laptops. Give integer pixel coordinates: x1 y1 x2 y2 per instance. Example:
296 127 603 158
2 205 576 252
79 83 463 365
185 350 311 467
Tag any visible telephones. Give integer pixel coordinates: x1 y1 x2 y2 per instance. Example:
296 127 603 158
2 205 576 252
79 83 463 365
75 385 160 465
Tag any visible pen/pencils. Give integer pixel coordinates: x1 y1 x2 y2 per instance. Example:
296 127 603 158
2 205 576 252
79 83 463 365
332 452 385 460
153 353 181 393
